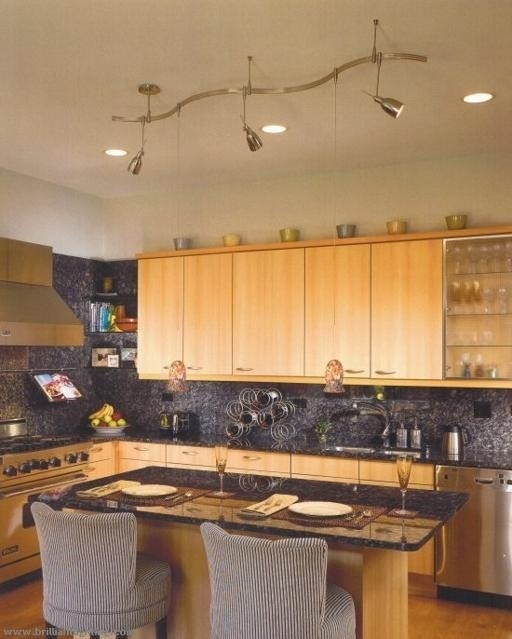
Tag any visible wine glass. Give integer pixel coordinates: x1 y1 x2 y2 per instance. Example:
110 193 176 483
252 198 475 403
394 455 413 515
214 439 229 496
447 241 511 377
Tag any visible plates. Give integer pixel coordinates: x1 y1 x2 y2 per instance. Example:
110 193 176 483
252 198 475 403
286 501 353 517
121 484 178 498
88 426 131 433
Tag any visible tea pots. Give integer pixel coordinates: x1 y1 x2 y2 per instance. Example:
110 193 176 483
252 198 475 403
439 422 472 456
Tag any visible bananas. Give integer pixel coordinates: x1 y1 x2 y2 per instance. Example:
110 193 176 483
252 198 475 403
88 403 114 420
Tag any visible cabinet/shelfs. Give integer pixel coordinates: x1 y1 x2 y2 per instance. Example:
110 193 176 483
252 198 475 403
222 447 290 478
133 248 232 382
360 458 434 574
84 294 138 374
166 442 220 471
230 241 304 384
119 438 165 475
445 225 512 389
303 232 444 388
289 453 361 487
84 442 117 480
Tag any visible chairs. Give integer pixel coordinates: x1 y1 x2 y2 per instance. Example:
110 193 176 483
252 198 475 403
28 499 177 639
192 517 362 638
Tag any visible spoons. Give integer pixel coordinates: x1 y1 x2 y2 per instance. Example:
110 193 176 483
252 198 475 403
173 490 195 503
344 509 374 524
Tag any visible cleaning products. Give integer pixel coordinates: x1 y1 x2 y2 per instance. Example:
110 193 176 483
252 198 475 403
454 353 473 377
90 302 116 332
476 355 485 377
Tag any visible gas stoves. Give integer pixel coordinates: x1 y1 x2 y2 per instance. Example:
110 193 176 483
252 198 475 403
0 436 95 490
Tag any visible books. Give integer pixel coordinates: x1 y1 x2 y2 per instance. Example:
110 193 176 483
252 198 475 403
89 300 115 332
33 374 82 401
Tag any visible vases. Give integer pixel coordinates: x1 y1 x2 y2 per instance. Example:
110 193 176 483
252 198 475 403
386 219 407 235
279 228 300 242
445 214 468 230
223 233 242 248
336 224 357 239
172 237 193 252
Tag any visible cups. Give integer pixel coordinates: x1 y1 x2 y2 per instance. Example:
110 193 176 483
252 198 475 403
102 276 112 293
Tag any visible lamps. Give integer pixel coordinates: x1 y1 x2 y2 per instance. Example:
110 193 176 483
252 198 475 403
109 18 432 176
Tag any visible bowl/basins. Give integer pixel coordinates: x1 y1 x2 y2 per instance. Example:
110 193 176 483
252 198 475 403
114 317 137 331
173 213 467 252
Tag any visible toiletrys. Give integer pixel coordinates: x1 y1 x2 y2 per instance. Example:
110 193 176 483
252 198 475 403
410 415 422 449
394 419 408 449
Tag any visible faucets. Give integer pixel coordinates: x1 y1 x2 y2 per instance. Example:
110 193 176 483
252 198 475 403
349 399 389 425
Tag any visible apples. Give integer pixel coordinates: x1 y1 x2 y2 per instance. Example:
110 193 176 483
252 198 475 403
112 411 124 420
98 422 107 428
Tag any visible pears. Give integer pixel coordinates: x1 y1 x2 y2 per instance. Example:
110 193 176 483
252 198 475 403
103 416 112 423
90 419 100 427
117 419 128 426
108 421 117 427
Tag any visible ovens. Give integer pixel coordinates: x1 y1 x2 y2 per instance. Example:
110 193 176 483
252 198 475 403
0 463 92 585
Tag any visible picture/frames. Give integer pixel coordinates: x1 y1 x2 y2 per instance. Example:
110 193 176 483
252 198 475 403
89 344 121 368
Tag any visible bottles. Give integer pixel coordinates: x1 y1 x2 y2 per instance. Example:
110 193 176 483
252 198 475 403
394 416 423 449
227 387 291 440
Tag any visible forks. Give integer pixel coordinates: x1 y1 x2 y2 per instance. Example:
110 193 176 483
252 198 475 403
253 497 283 512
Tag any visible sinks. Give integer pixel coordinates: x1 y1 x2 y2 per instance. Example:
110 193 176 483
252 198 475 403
371 449 420 460
324 446 377 456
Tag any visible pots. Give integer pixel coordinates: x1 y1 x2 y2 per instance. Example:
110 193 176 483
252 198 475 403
0 417 27 442
